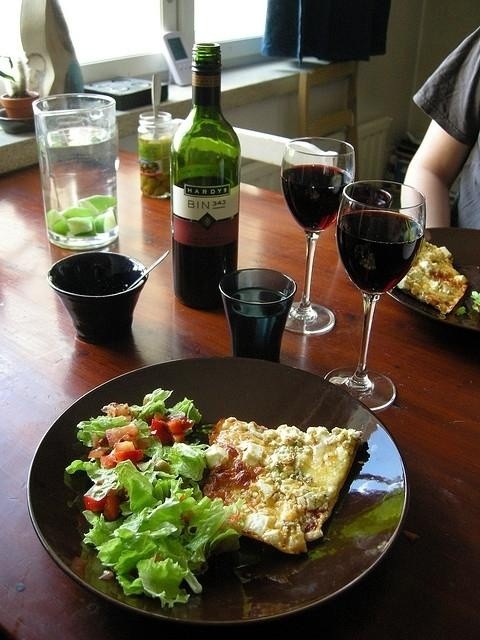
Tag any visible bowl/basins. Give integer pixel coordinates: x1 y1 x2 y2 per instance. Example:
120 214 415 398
0 108 34 135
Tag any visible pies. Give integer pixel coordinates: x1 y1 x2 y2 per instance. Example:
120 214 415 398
399 241 467 314
202 415 362 554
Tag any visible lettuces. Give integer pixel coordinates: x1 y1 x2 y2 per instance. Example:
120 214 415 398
65 389 229 596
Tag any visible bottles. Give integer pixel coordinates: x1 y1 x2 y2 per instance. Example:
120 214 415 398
136 111 174 200
169 41 239 312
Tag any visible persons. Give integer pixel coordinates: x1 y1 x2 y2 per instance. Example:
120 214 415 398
398 23 480 231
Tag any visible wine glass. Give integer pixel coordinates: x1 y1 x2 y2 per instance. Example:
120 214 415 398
322 180 424 417
279 138 356 333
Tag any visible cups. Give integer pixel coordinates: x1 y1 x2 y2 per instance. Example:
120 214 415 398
45 244 150 343
31 92 120 251
219 266 297 367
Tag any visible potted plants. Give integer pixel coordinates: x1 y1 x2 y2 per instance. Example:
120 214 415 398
0 57 39 118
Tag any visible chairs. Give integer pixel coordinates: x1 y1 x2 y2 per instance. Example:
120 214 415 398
172 118 338 196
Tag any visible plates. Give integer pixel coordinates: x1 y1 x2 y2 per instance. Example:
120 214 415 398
384 225 480 332
28 354 410 624
